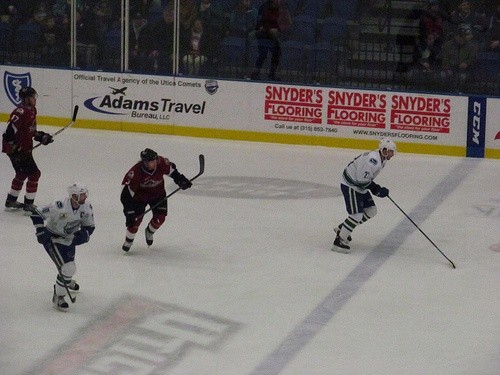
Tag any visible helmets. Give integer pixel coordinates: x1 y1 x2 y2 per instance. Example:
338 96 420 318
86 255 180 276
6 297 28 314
379 137 396 157
18 87 38 98
68 182 88 202
140 148 158 161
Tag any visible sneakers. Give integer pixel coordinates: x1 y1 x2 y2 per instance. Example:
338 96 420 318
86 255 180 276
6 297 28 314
4 194 24 211
331 237 351 253
333 225 352 241
22 196 39 216
68 280 80 302
145 225 155 248
52 294 69 311
122 237 134 254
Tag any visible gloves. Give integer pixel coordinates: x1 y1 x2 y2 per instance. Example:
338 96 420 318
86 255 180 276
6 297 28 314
72 229 91 245
174 174 193 191
124 207 135 229
35 131 54 145
371 184 389 198
36 227 49 243
15 145 28 160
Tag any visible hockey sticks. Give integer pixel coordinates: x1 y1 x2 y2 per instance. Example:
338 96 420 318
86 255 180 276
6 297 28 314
387 195 456 269
138 153 205 219
26 104 79 153
47 243 77 304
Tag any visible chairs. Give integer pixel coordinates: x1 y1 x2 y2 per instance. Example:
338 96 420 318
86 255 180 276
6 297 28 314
101 0 360 82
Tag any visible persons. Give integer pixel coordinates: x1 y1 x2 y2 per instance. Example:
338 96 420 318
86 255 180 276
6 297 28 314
332 138 396 254
1 0 500 98
1 88 54 213
32 183 95 312
120 149 193 254
